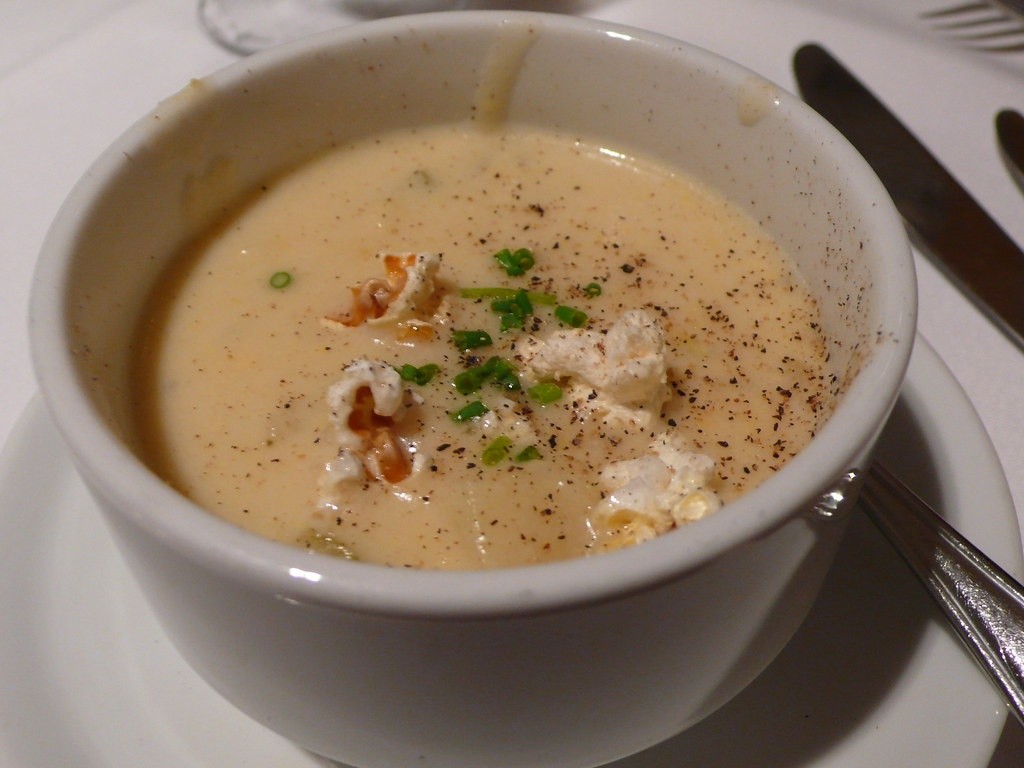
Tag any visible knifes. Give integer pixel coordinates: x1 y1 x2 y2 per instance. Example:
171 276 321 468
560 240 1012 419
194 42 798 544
996 110 1024 199
795 43 1024 348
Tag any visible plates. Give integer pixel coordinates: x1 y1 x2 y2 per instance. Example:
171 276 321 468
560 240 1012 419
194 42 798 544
0 319 1024 768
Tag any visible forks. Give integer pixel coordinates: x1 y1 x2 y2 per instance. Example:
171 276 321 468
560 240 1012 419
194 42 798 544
918 0 1024 54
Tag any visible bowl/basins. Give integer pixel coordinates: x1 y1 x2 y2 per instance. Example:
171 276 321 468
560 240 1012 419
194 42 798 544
27 10 919 768
